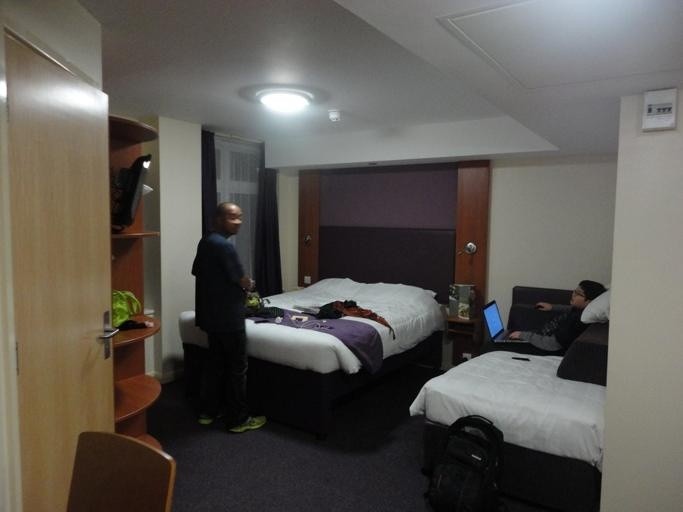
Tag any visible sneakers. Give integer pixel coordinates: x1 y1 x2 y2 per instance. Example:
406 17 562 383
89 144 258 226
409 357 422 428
197 412 223 425
227 415 267 434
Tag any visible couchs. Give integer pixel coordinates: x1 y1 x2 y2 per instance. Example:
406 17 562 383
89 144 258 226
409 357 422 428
424 287 609 512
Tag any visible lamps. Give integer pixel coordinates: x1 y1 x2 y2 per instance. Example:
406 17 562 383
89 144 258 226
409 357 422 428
254 87 316 117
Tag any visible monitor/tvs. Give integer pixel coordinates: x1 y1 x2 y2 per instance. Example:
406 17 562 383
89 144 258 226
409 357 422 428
112 154 152 232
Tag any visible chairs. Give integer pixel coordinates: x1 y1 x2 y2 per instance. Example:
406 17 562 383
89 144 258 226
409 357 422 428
67 432 176 512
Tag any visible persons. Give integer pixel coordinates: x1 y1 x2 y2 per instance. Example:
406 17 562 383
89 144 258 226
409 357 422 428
470 279 606 360
189 201 270 435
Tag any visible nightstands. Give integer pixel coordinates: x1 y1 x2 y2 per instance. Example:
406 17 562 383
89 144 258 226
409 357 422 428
445 315 484 359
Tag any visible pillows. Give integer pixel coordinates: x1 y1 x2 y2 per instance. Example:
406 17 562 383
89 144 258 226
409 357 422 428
579 289 610 325
302 276 437 316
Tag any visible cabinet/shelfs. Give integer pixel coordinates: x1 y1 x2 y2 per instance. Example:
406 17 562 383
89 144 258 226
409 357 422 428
109 113 163 451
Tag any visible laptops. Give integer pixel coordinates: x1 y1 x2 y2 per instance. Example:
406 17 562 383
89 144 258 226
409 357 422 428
481 300 529 343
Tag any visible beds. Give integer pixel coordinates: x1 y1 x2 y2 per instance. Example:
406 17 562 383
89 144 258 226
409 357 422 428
178 225 457 436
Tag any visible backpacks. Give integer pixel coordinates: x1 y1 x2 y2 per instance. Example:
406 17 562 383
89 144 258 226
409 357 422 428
423 412 511 512
